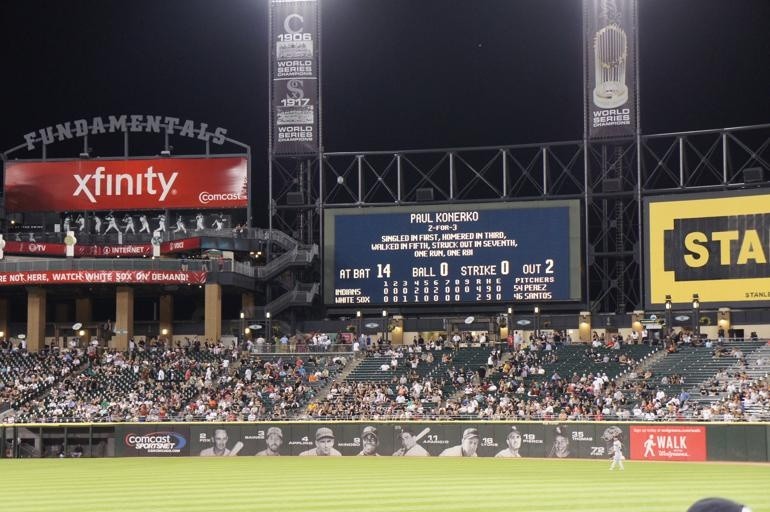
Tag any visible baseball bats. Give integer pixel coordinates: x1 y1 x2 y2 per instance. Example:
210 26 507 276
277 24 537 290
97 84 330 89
228 441 244 456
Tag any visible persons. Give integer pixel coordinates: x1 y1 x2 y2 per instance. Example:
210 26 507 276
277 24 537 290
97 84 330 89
356 426 382 456
255 427 285 455
300 427 342 456
180 253 227 272
61 212 227 237
1 318 769 422
494 427 522 457
546 426 576 457
608 435 625 470
58 442 83 458
438 427 480 457
200 427 237 456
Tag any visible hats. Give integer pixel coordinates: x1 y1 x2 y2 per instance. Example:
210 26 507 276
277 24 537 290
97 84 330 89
267 427 282 439
463 428 481 440
687 498 744 512
552 426 568 438
505 425 521 436
316 427 335 440
361 426 378 440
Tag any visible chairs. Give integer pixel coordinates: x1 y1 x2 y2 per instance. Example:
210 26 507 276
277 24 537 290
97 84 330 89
0 340 769 424
